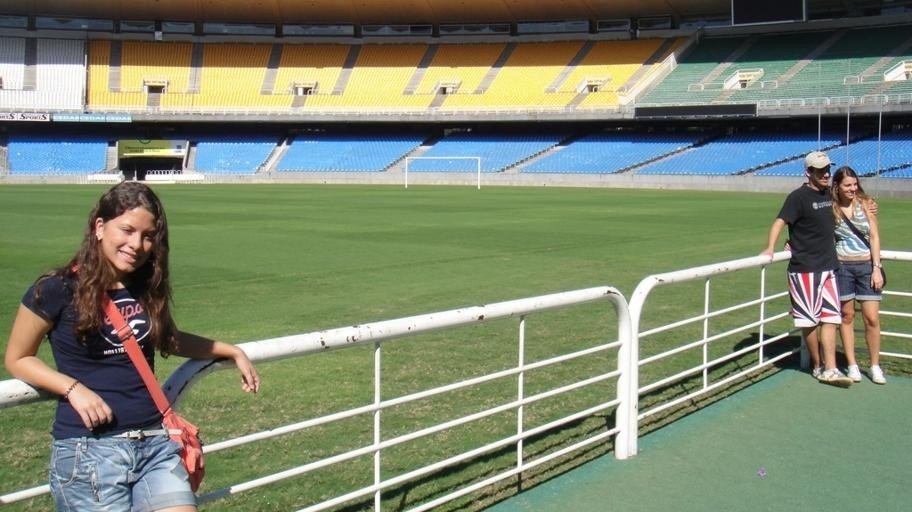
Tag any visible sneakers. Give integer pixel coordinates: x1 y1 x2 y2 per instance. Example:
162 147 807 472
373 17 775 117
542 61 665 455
817 368 854 388
847 364 862 382
812 367 825 378
867 364 887 385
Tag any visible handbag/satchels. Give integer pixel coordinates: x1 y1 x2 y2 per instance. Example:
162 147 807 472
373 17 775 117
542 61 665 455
880 266 887 287
161 411 205 492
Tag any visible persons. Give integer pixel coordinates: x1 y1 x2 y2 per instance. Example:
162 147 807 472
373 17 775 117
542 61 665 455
758 150 880 386
783 165 887 384
3 178 261 512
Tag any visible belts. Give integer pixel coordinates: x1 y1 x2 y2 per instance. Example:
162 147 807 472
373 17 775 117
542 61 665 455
113 429 183 439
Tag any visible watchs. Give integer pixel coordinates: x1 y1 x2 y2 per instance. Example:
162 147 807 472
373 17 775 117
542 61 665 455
872 262 884 269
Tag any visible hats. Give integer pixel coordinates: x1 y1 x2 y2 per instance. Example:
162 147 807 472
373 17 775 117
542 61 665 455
803 151 836 171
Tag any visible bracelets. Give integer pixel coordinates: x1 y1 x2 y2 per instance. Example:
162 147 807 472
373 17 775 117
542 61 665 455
63 378 84 401
